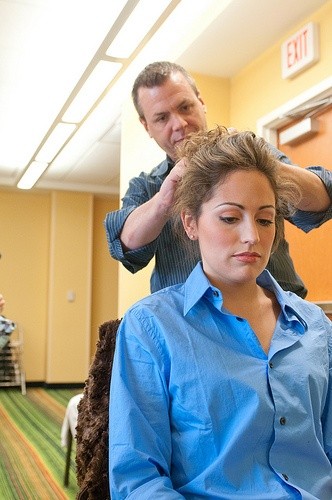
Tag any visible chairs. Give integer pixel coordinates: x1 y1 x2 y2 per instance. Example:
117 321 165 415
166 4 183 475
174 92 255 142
57 392 85 488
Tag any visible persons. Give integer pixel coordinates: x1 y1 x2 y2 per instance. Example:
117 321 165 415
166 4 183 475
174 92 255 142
105 62 332 300
110 127 332 500
